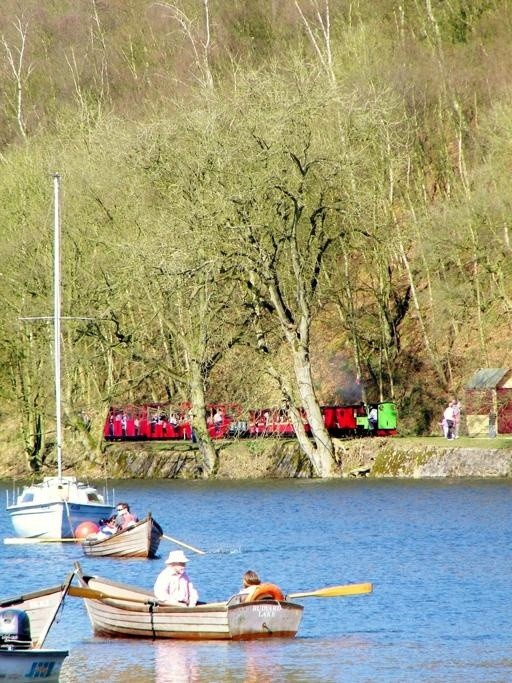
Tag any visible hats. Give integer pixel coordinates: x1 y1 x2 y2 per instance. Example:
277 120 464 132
165 551 190 563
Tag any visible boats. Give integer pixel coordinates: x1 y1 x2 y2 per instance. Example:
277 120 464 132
73 510 168 560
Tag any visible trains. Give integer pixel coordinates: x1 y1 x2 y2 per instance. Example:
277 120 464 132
98 395 402 440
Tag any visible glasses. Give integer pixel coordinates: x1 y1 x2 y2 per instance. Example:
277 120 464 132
115 507 127 512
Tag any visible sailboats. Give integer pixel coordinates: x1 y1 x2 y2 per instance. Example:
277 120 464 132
5 167 120 549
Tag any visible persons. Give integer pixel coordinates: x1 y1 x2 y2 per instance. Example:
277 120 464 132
452 399 461 439
112 502 137 536
228 569 263 606
443 400 455 440
366 404 378 432
437 414 449 437
152 549 200 608
88 514 119 542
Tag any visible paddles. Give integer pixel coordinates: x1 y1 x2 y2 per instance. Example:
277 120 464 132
4 537 94 545
68 585 176 606
287 583 373 599
